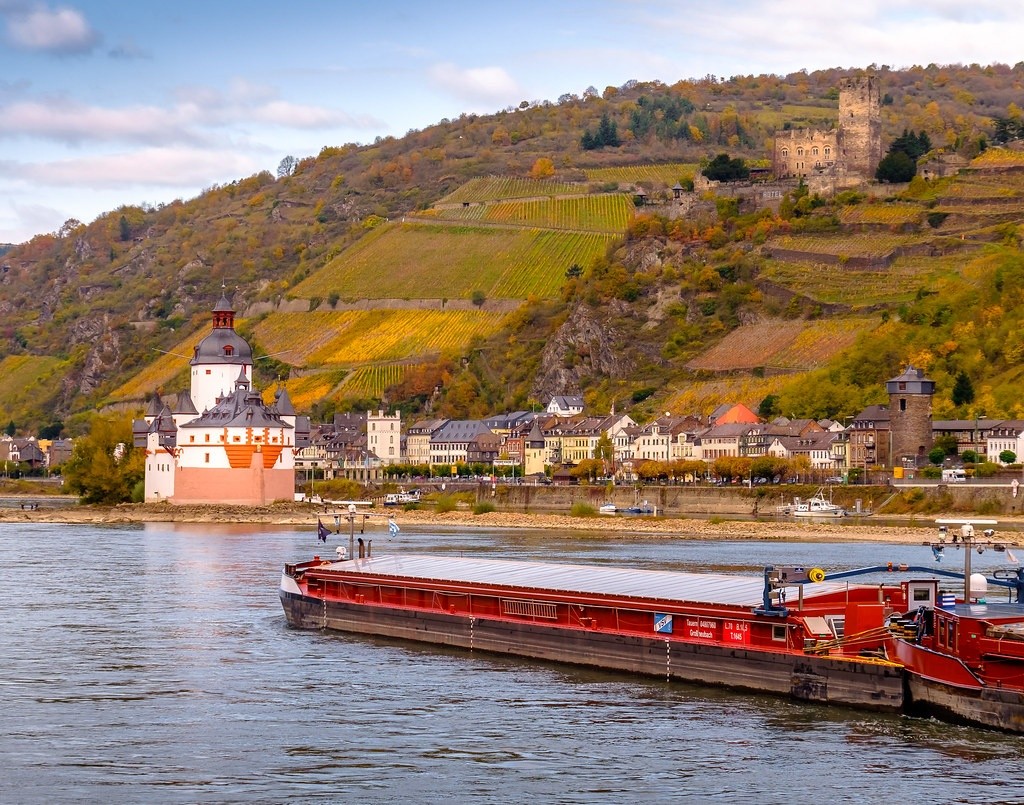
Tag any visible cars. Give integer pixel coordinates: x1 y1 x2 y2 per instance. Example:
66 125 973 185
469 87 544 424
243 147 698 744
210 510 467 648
787 478 796 484
649 472 780 485
427 472 523 485
837 476 844 484
825 477 838 484
594 477 612 484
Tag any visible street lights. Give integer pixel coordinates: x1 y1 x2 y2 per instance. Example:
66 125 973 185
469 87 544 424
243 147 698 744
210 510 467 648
345 503 357 560
920 525 1020 603
666 410 670 466
748 468 752 489
861 443 875 485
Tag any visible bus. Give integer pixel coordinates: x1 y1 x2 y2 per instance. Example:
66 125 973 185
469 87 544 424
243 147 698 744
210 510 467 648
942 468 967 485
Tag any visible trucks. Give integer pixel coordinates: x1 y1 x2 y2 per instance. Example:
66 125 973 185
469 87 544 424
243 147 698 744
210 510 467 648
538 477 559 484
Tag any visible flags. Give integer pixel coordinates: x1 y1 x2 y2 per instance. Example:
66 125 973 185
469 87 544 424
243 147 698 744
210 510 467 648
389 520 399 537
318 519 331 541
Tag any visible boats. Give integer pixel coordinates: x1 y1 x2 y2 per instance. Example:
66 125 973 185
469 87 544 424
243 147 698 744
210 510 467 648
598 504 620 513
884 595 1024 741
777 485 845 519
279 549 964 709
623 486 652 513
383 488 422 506
